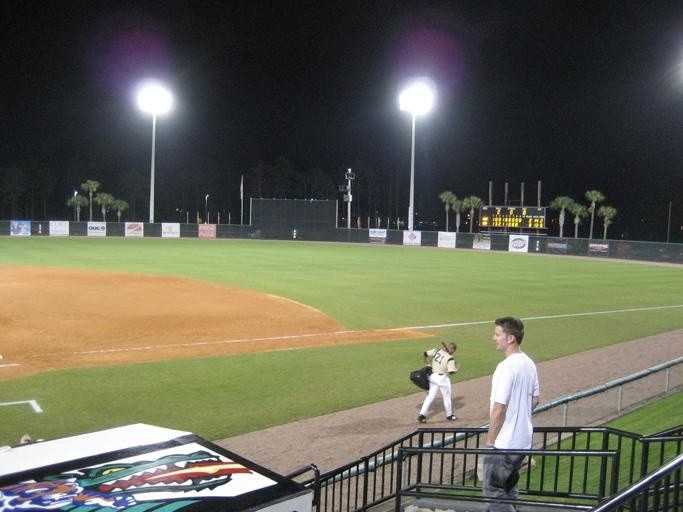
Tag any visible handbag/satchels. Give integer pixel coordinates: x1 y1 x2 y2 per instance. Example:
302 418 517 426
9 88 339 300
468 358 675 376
411 366 432 390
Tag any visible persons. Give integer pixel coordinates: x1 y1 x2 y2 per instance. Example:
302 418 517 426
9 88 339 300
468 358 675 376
416 340 462 422
480 316 540 511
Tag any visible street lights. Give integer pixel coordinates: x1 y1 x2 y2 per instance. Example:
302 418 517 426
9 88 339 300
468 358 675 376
397 75 437 231
135 76 177 222
345 168 357 228
73 191 78 220
205 194 211 224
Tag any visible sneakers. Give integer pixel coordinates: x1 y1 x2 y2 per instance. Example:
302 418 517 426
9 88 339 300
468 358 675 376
417 414 427 424
447 415 457 420
